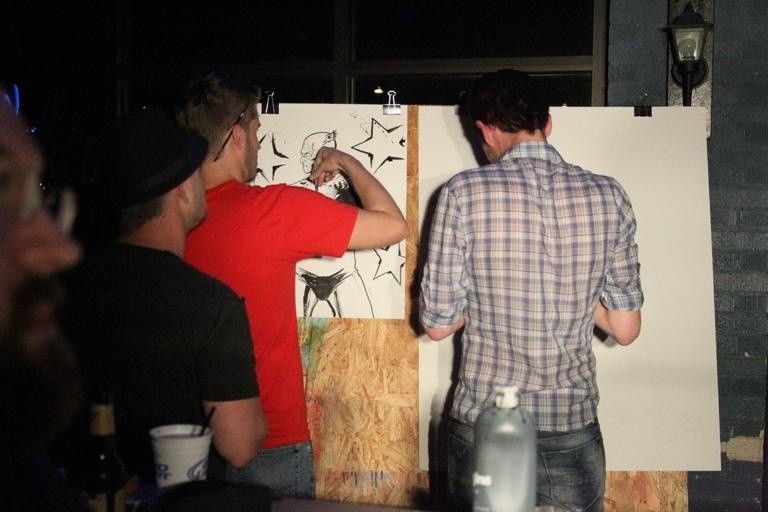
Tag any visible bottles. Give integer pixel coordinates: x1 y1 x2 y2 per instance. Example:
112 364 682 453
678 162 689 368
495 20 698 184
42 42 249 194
472 384 538 512
72 374 128 512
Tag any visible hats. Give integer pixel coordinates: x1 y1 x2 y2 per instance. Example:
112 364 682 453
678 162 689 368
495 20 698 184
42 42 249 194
85 103 211 211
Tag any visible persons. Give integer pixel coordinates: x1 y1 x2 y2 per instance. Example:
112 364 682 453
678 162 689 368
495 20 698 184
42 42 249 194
35 99 269 487
1 90 271 509
411 71 644 512
173 56 407 499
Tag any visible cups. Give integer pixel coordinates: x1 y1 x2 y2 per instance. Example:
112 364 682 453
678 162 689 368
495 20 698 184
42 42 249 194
149 423 214 490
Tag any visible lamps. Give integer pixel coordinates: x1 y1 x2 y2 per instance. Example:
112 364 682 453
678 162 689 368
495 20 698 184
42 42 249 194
662 1 713 109
